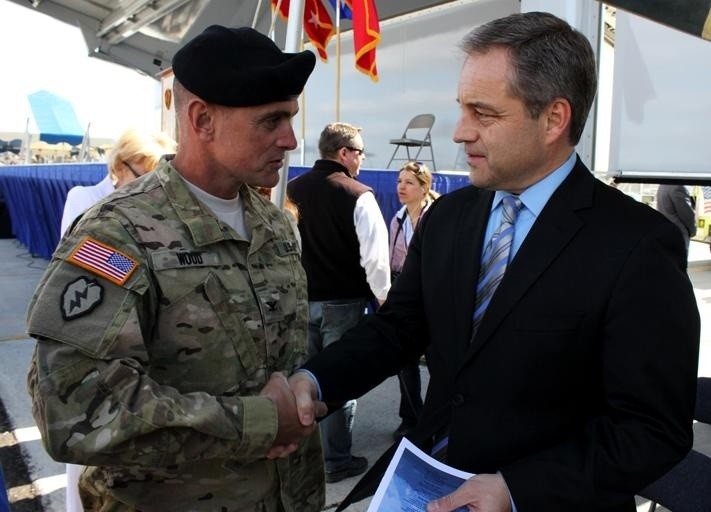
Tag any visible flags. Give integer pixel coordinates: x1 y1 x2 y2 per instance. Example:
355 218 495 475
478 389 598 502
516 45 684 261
327 0 382 83
267 0 337 64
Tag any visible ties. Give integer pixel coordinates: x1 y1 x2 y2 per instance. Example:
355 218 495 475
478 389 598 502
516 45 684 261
469 196 524 343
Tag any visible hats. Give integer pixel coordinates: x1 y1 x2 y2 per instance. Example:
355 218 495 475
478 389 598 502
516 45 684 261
172 25 317 107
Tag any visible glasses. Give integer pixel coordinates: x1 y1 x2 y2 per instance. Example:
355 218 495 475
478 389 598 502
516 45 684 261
124 159 141 181
339 147 365 158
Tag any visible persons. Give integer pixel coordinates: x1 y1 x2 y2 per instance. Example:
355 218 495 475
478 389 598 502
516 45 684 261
285 122 394 483
22 22 330 511
656 182 698 257
57 130 165 242
267 12 701 512
391 158 445 445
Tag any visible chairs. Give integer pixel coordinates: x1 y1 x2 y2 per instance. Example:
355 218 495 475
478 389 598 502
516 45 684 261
385 114 436 173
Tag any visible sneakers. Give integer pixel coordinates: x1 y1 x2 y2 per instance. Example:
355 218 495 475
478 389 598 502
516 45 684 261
325 455 368 483
